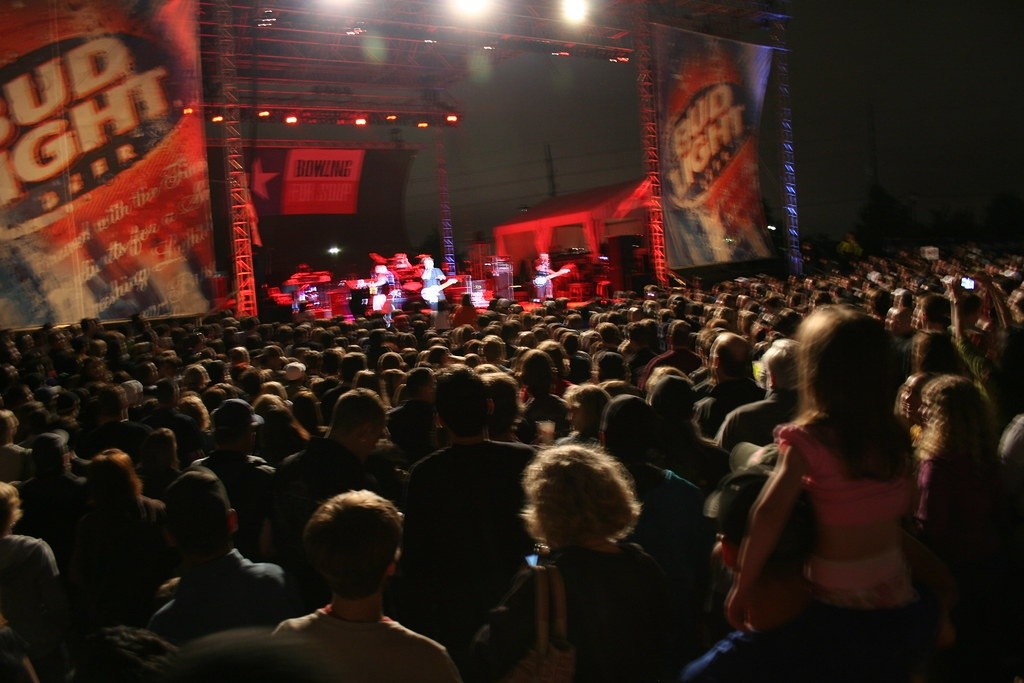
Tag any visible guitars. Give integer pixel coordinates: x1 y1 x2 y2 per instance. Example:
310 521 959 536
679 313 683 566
420 278 458 301
533 268 571 289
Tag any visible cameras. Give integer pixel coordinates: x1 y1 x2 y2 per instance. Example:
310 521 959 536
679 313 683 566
959 277 975 290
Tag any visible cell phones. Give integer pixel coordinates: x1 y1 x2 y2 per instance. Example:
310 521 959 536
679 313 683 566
522 551 539 567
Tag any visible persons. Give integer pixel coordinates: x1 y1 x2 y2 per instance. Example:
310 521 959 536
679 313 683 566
0 230 1024 683
